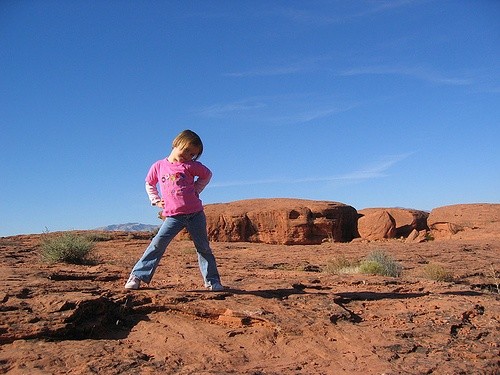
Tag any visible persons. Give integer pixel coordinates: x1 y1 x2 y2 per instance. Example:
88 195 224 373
124 129 226 291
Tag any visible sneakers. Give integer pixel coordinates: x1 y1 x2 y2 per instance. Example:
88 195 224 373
124 275 141 289
208 281 225 291
